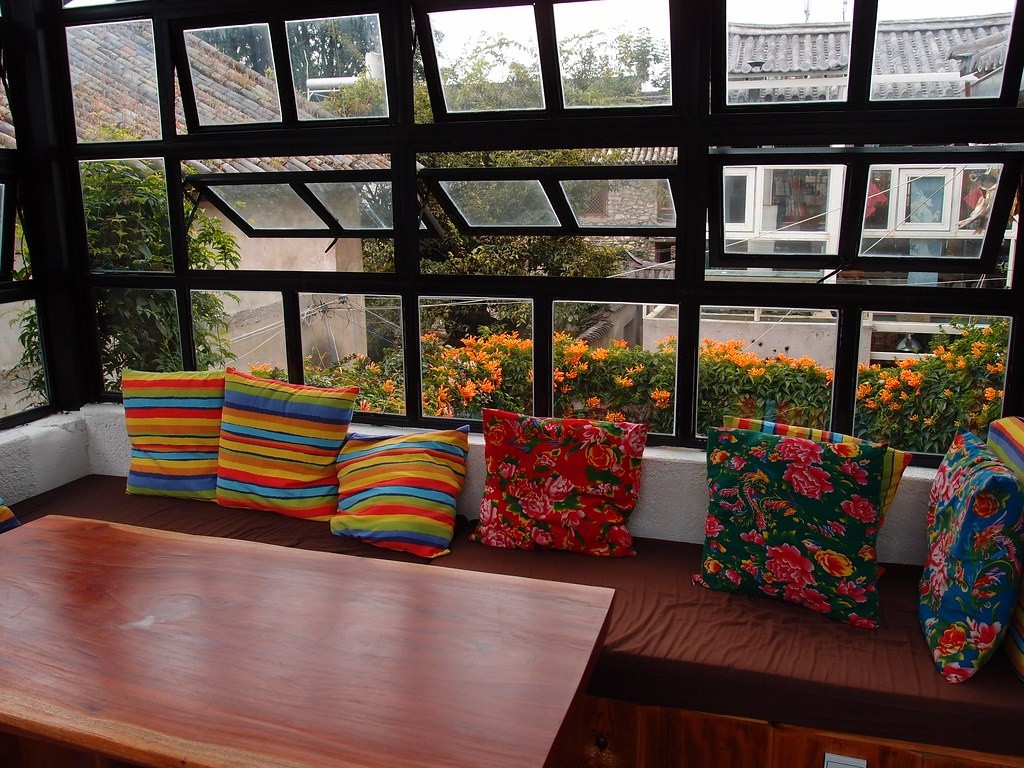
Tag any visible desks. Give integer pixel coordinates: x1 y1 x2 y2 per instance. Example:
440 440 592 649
0 514 618 768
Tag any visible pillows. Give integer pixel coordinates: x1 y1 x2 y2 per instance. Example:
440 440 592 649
122 368 228 503
917 424 1023 685
693 426 890 630
331 425 469 558
722 416 912 581
0 497 20 533
987 415 1023 678
468 408 648 557
212 366 360 521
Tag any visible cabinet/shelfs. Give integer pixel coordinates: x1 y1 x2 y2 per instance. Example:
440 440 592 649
558 696 1023 768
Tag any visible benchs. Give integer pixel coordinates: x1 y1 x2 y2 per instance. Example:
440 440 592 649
0 473 1023 759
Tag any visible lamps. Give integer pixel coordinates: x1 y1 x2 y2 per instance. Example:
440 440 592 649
896 332 923 352
